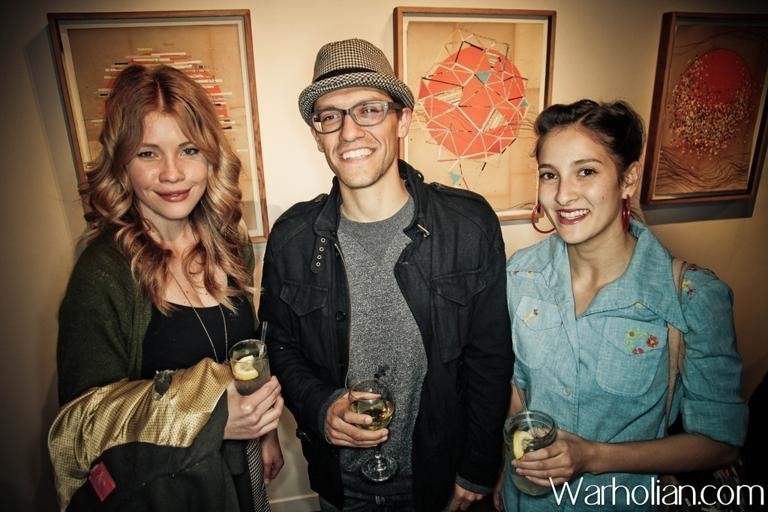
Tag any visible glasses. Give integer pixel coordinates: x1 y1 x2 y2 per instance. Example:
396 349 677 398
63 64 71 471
308 100 406 134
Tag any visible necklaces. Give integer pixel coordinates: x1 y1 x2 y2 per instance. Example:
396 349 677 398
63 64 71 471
161 260 233 367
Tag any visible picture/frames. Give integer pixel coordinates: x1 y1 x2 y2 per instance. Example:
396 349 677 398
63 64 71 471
392 7 557 222
640 11 767 208
47 9 271 243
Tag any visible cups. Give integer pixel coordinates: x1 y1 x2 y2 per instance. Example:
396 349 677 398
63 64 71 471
501 408 556 497
228 340 273 412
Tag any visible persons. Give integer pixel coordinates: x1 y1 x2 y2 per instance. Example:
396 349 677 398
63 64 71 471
491 97 749 512
55 64 285 511
257 36 512 512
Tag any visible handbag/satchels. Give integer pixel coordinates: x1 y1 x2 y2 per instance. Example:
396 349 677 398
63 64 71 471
656 413 752 511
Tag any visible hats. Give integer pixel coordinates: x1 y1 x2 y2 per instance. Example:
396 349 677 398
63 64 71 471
299 39 415 127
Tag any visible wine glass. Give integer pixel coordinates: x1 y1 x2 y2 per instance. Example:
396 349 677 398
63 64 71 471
348 379 397 482
